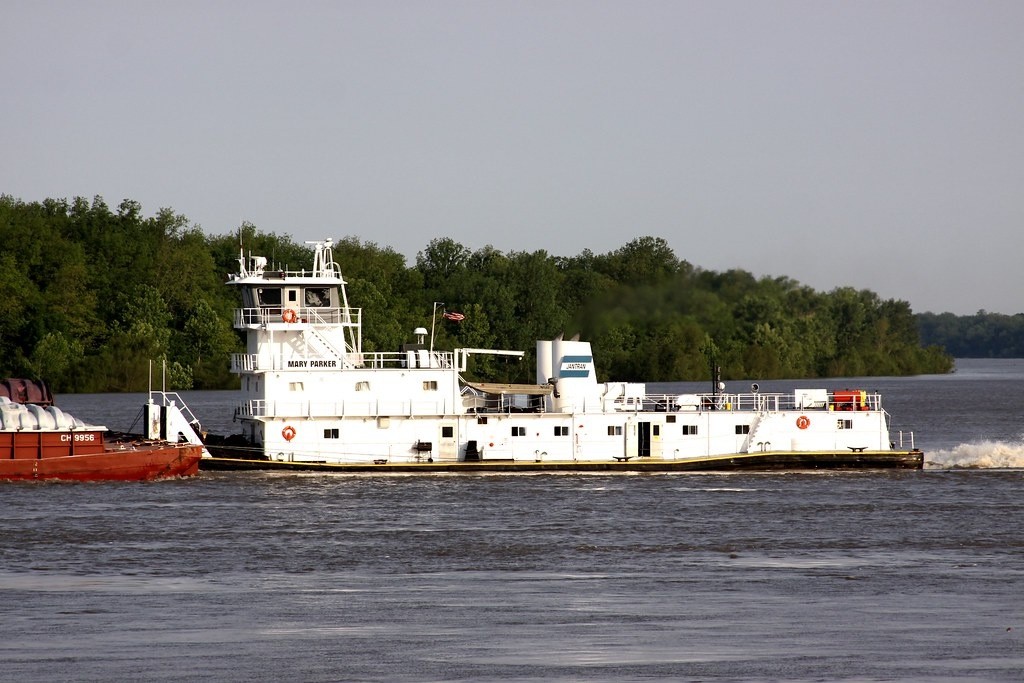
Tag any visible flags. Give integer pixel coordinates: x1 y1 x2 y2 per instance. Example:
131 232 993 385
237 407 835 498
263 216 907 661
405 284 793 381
443 310 465 321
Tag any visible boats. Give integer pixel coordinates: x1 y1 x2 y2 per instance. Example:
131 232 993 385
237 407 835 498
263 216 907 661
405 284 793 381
144 220 927 474
0 355 213 485
464 376 562 399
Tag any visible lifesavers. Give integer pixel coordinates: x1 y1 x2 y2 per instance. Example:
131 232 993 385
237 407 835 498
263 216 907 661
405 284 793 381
282 309 296 322
283 426 296 440
797 416 810 430
